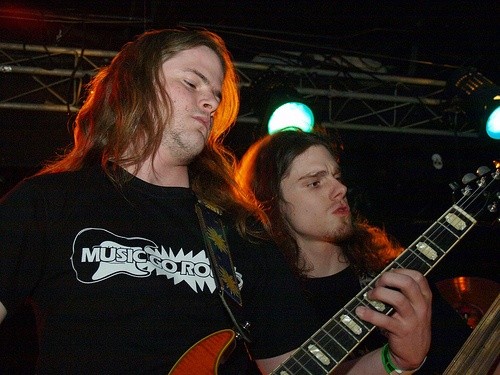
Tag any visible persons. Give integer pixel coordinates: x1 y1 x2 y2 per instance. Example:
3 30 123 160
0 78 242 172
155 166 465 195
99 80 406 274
454 304 483 331
0 25 431 375
235 127 476 374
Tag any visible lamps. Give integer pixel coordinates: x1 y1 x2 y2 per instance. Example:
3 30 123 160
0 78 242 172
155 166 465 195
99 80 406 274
446 59 500 140
247 69 316 137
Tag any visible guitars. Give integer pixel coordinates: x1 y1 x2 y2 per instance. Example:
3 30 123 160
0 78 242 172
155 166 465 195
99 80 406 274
169 166 500 375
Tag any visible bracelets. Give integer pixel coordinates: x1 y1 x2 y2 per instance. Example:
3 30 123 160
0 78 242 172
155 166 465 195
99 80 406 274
380 342 428 374
